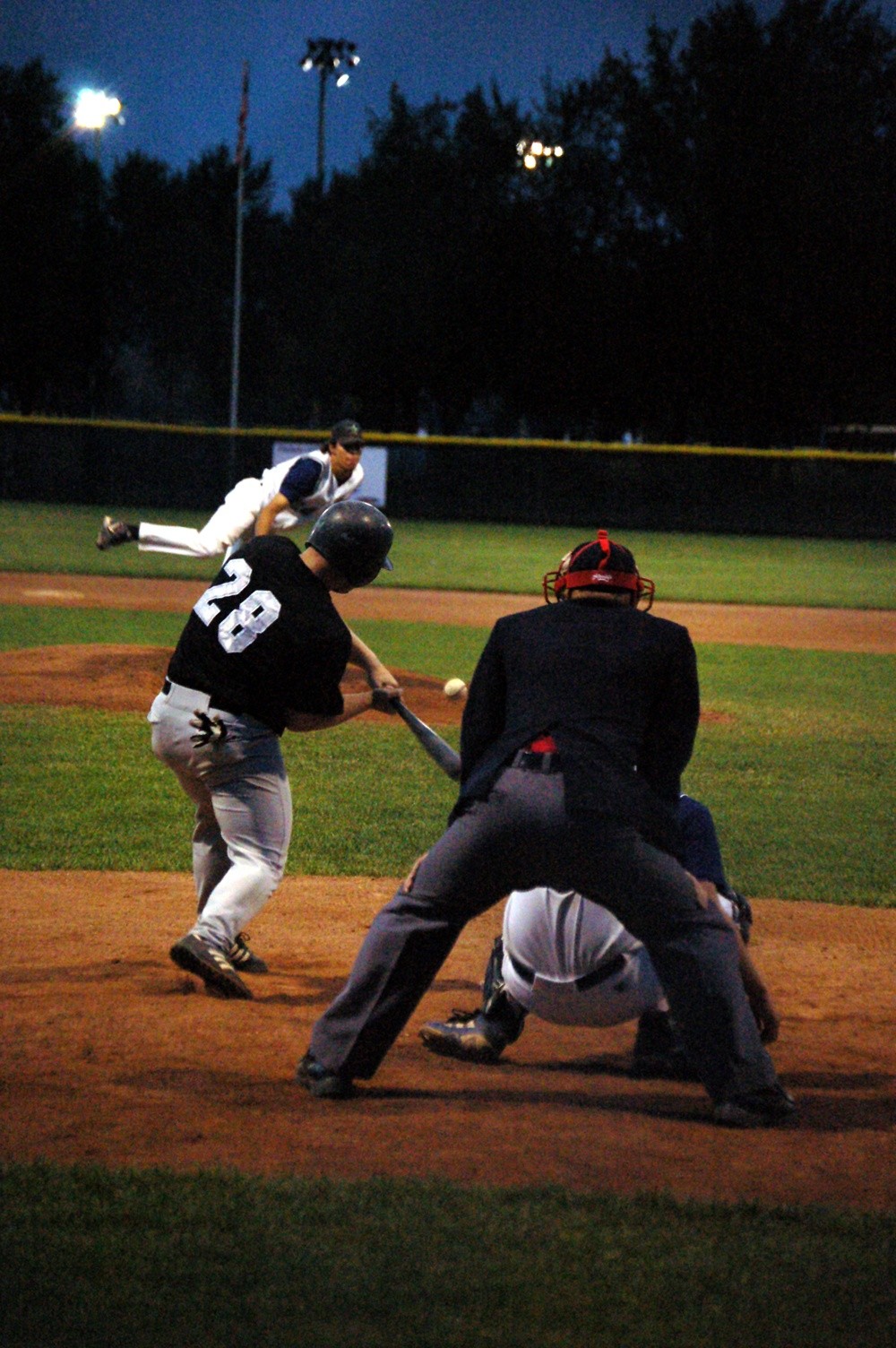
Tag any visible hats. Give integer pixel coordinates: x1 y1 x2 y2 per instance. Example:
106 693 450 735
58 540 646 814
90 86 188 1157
332 419 365 448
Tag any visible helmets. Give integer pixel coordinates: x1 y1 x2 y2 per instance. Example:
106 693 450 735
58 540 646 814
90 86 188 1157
542 529 656 612
302 498 398 587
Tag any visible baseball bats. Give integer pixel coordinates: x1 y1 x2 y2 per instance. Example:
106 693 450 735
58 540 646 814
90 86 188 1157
398 702 460 782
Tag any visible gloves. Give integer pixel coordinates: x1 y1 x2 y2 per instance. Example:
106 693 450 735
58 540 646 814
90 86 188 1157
189 709 229 749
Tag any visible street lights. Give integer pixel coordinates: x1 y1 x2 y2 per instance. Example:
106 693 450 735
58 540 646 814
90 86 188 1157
299 33 361 186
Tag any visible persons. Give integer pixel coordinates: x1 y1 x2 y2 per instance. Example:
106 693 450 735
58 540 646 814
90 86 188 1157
94 417 366 569
410 787 741 1083
142 501 406 999
297 528 800 1128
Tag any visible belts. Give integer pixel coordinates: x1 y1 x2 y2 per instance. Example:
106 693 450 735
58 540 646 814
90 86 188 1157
509 955 631 990
161 680 243 720
507 750 577 774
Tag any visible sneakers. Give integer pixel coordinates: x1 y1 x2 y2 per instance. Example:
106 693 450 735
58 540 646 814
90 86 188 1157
97 513 131 551
415 1005 501 1068
223 932 271 977
169 931 255 1002
705 1083 803 1127
295 1048 358 1102
623 1041 696 1081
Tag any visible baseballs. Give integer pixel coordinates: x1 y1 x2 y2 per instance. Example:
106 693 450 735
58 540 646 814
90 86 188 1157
441 678 467 703
